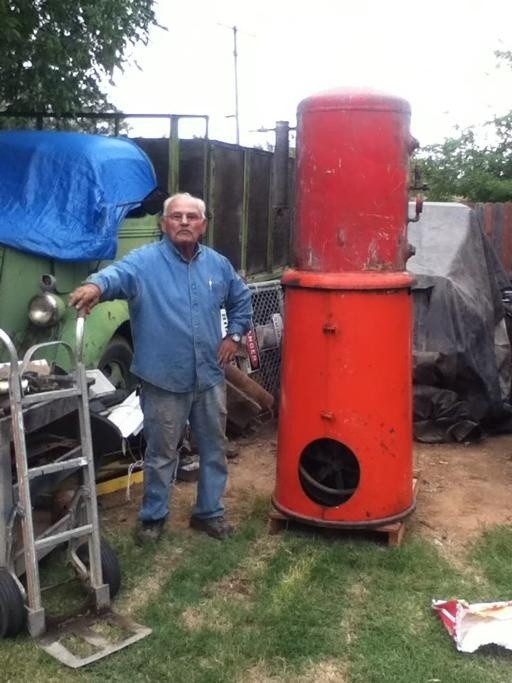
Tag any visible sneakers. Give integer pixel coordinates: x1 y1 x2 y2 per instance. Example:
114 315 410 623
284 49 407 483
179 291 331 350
136 519 165 546
189 514 237 540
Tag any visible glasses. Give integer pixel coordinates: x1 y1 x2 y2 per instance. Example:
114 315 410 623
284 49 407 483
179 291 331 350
163 213 202 221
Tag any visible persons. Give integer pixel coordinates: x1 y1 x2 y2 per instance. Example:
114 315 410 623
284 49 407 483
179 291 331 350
67 191 254 546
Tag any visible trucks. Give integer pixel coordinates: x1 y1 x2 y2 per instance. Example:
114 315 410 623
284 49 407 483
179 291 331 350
0 111 294 398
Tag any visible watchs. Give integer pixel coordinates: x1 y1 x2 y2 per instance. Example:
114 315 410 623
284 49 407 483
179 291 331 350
228 331 241 344
